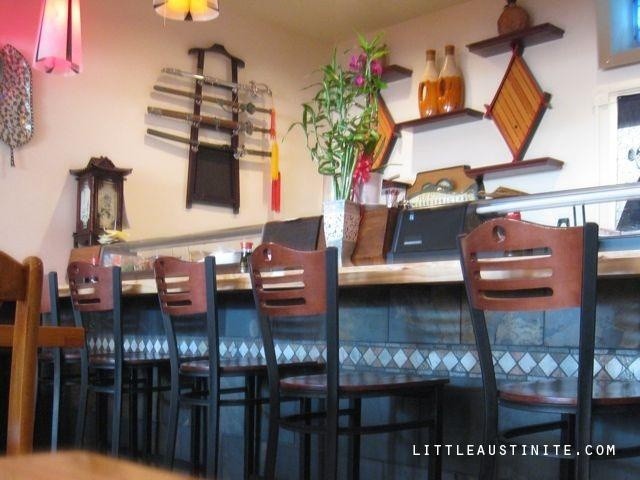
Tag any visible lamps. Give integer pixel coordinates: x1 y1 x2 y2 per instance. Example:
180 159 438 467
32 1 86 76
153 1 223 27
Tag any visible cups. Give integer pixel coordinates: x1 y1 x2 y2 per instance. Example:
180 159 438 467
385 190 398 209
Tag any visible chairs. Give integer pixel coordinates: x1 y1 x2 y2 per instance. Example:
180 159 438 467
0 214 640 480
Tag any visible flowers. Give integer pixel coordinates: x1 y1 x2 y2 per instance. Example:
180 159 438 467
282 26 392 203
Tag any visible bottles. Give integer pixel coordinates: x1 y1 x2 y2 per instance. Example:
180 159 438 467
505 211 522 257
240 241 253 272
90 257 100 283
497 0 529 35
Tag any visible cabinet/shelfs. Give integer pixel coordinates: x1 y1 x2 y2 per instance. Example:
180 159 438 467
344 21 568 196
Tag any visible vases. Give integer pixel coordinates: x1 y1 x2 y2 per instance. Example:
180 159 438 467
323 200 360 268
